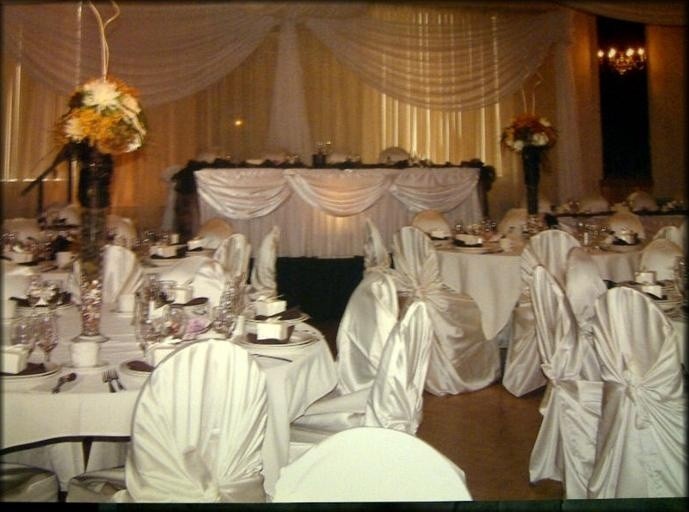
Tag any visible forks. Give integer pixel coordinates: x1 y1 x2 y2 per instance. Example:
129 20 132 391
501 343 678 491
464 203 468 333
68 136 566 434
103 368 125 393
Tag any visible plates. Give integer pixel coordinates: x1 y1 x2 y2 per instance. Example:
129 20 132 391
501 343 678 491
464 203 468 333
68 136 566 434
60 360 109 371
0 362 61 379
245 311 313 324
430 236 647 253
15 302 73 312
120 359 152 377
111 308 134 318
233 329 316 350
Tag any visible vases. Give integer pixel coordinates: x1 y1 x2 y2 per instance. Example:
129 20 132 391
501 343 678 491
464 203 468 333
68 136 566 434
520 152 542 216
76 151 113 342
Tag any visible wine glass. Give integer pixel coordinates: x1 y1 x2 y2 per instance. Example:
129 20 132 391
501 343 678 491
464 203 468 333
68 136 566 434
10 273 68 362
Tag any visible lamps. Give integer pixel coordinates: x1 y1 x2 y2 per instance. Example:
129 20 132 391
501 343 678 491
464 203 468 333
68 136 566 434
596 46 648 73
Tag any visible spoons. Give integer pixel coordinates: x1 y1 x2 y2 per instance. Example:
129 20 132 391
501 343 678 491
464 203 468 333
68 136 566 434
51 372 77 393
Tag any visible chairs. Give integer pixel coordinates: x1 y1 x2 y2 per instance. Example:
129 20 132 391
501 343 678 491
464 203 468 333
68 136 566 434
364 208 503 398
1 203 280 307
65 338 270 504
288 299 434 462
272 426 473 502
318 269 398 402
0 462 61 505
497 189 688 501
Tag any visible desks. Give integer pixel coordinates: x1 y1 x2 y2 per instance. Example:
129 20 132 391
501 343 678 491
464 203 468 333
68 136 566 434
3 307 324 454
161 159 494 261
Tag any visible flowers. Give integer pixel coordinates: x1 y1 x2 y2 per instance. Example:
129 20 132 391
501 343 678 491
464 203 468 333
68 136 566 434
56 75 149 155
500 111 558 154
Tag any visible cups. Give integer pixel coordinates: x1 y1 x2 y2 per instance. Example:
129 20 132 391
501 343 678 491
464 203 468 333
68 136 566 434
56 252 78 269
71 340 101 366
134 228 243 361
118 295 134 313
454 217 609 246
